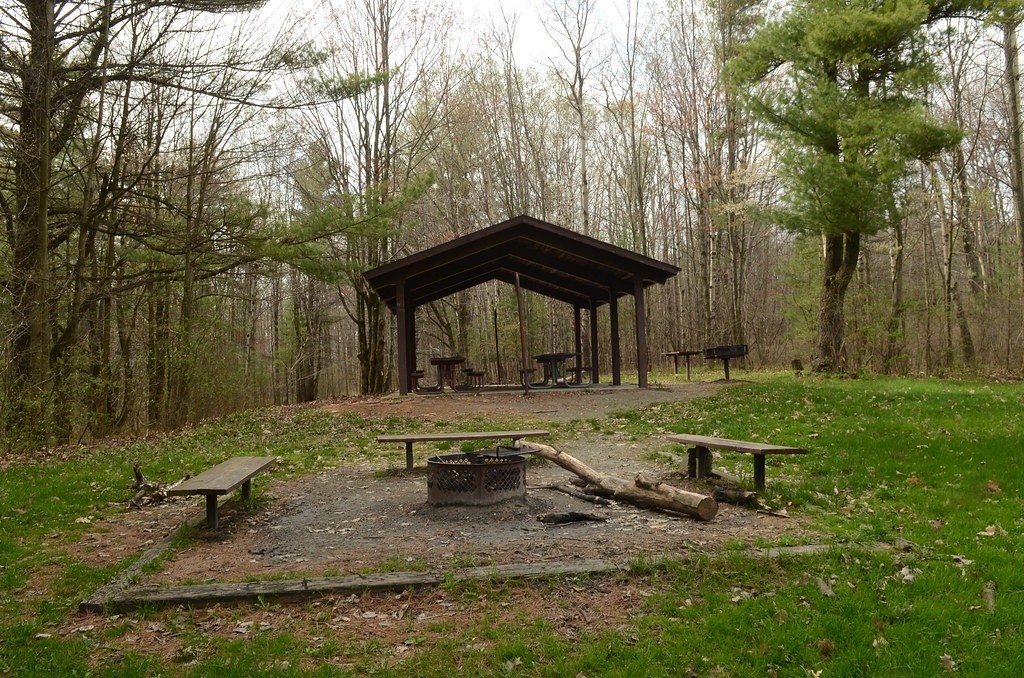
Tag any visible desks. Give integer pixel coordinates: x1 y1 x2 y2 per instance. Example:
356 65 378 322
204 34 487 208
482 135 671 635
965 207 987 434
661 350 704 382
532 352 581 388
429 355 466 393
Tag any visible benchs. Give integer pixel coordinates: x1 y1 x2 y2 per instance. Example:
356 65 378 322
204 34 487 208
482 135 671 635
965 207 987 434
667 433 809 491
376 430 550 471
166 457 277 532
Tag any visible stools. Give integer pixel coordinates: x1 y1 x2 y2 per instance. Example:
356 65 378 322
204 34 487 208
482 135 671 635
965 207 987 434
411 365 593 393
